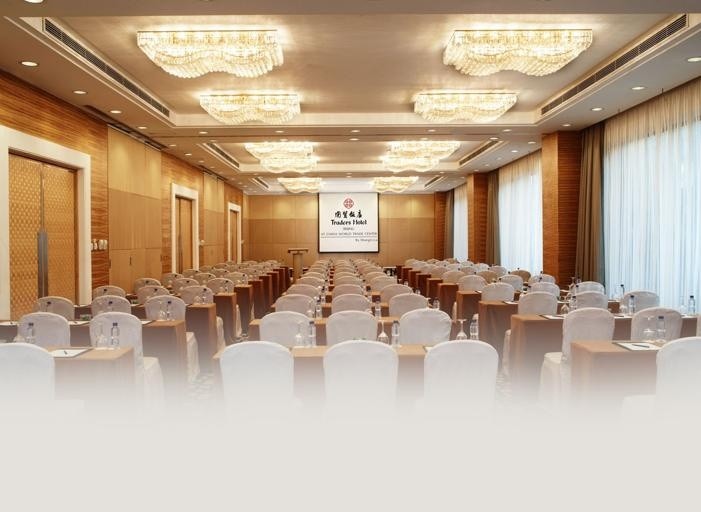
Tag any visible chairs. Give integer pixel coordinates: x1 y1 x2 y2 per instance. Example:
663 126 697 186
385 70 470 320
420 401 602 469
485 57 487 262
0 240 701 404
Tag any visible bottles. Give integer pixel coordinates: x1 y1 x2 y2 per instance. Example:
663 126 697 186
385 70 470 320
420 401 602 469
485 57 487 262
24 260 277 349
296 258 481 344
427 259 696 341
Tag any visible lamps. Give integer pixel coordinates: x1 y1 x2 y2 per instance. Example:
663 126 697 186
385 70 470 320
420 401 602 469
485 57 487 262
444 27 598 81
243 142 319 174
381 142 461 173
372 176 420 193
199 94 300 125
276 177 322 194
134 28 286 83
412 90 519 124
